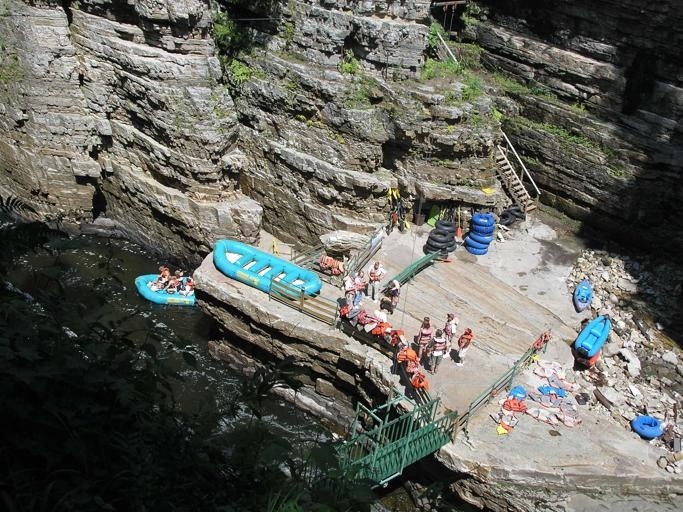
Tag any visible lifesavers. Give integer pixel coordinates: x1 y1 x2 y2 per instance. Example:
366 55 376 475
464 214 495 255
423 220 456 260
631 416 663 437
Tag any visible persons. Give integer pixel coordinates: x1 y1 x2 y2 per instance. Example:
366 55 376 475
366 261 384 302
373 303 387 323
425 329 446 375
415 316 432 362
147 265 194 298
455 328 473 366
441 312 459 358
352 269 366 309
342 274 356 307
386 279 401 308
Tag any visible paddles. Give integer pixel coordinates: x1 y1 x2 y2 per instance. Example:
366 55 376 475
457 207 462 239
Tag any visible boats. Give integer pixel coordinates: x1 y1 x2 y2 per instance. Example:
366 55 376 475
134 273 197 306
212 238 321 298
574 315 611 357
572 278 592 313
